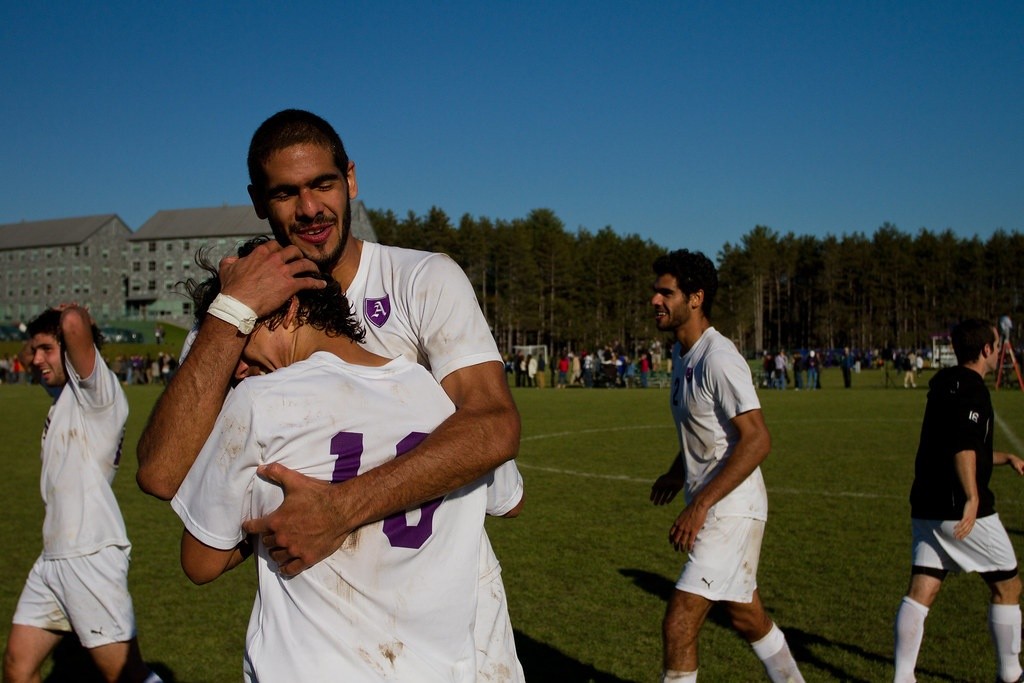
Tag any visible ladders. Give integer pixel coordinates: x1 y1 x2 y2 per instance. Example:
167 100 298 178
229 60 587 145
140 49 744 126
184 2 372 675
995 339 1024 392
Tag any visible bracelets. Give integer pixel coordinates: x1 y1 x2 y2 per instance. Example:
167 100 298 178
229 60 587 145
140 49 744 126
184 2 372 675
206 292 258 337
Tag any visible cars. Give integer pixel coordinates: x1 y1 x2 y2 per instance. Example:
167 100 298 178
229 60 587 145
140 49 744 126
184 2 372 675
100 327 144 345
0 323 22 344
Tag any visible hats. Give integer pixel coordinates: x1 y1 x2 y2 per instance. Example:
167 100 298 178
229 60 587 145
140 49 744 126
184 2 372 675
810 350 815 358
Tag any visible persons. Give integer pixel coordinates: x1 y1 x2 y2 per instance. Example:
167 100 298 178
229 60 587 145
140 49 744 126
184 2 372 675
496 341 674 391
642 249 810 683
0 309 178 390
0 305 179 683
887 317 1024 683
749 345 948 392
997 315 1012 338
135 108 529 683
174 230 529 683
